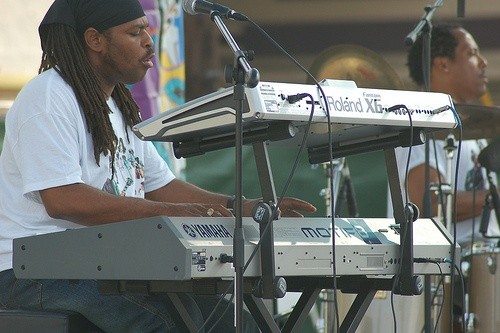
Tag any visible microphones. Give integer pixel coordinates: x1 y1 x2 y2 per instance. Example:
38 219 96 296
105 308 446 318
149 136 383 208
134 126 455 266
182 0 248 22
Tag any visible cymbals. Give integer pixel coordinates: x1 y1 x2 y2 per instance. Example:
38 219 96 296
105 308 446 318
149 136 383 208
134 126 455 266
307 45 404 90
478 141 500 172
424 104 500 141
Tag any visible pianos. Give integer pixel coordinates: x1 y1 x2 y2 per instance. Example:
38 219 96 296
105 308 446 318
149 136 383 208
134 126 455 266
12 215 461 280
133 81 459 147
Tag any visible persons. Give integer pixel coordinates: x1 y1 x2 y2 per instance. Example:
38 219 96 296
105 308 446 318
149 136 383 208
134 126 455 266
386 22 500 246
0 0 317 333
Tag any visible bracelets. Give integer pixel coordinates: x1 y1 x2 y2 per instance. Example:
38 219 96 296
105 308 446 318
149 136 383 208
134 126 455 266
227 194 246 217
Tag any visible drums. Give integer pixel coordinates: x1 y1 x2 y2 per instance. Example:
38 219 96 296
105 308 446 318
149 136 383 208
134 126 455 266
325 273 451 333
462 247 500 333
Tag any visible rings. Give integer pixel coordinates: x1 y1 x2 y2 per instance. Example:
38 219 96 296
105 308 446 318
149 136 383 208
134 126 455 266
206 208 214 217
